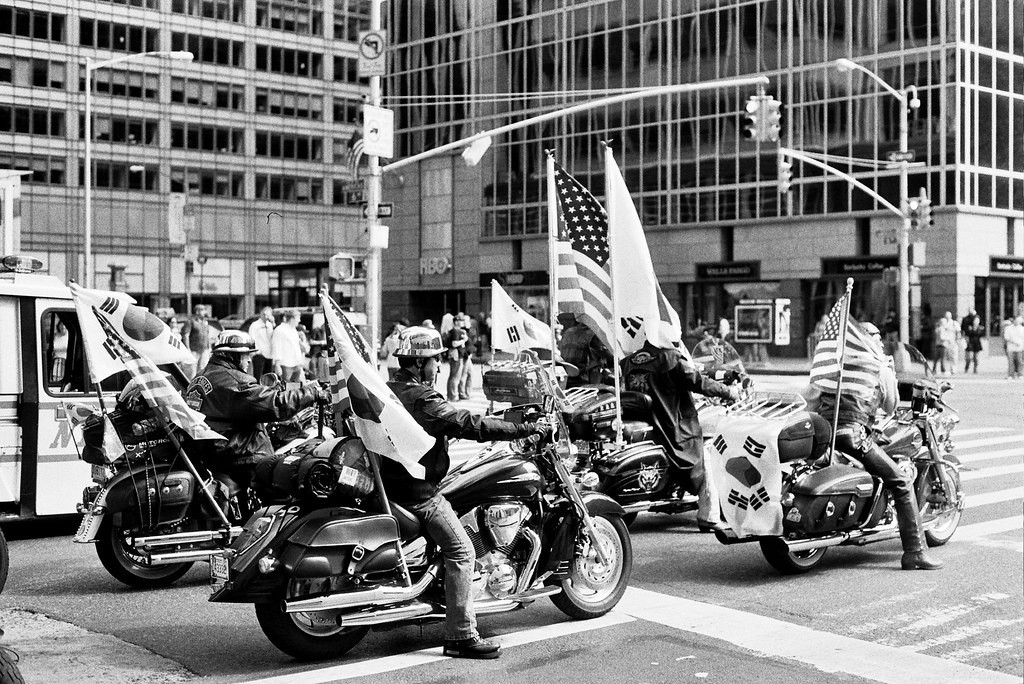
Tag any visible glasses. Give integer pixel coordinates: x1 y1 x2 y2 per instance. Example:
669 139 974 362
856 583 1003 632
431 355 441 362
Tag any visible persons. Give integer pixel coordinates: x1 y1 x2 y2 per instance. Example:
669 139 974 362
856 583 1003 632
715 314 731 342
50 320 69 382
380 311 492 402
186 329 334 491
884 309 1024 380
812 322 946 571
167 304 311 382
370 325 555 661
558 322 614 390
619 338 738 533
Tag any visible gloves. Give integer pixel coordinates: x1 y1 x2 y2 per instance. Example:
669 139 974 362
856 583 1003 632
519 422 554 441
318 389 334 405
728 384 740 400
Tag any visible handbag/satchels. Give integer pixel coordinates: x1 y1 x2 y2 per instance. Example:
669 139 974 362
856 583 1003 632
447 349 459 363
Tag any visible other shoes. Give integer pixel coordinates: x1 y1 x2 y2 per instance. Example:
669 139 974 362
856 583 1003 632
930 368 1023 383
901 549 944 570
697 518 732 532
459 394 470 400
447 396 459 402
443 635 502 658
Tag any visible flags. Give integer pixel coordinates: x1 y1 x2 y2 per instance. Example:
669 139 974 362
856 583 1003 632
319 293 436 479
101 412 125 463
810 294 886 401
546 154 648 361
490 279 562 356
710 412 789 538
62 401 102 431
68 280 198 383
549 367 575 415
606 146 707 375
91 305 229 441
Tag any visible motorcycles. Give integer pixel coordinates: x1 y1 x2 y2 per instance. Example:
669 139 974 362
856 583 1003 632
713 345 965 574
72 372 335 589
208 347 632 661
481 336 755 526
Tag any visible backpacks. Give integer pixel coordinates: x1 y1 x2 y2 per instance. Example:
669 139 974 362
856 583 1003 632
180 318 194 344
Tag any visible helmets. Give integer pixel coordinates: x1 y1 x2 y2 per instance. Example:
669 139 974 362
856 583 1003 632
392 325 449 357
209 330 260 352
859 322 880 335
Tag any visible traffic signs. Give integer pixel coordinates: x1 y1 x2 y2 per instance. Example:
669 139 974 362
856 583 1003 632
362 203 393 219
885 151 915 162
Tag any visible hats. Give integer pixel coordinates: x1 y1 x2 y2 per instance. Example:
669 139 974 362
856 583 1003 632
422 319 436 329
453 312 466 323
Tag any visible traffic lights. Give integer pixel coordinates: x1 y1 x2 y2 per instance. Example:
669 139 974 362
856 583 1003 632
881 268 899 283
910 268 919 284
906 197 921 230
744 100 761 140
778 163 794 195
765 100 782 143
920 200 934 232
330 255 354 282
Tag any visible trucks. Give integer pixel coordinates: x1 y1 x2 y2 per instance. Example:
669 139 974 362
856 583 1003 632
0 272 134 526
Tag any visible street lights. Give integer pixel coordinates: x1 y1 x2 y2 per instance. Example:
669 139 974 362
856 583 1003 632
84 50 194 288
835 59 912 373
